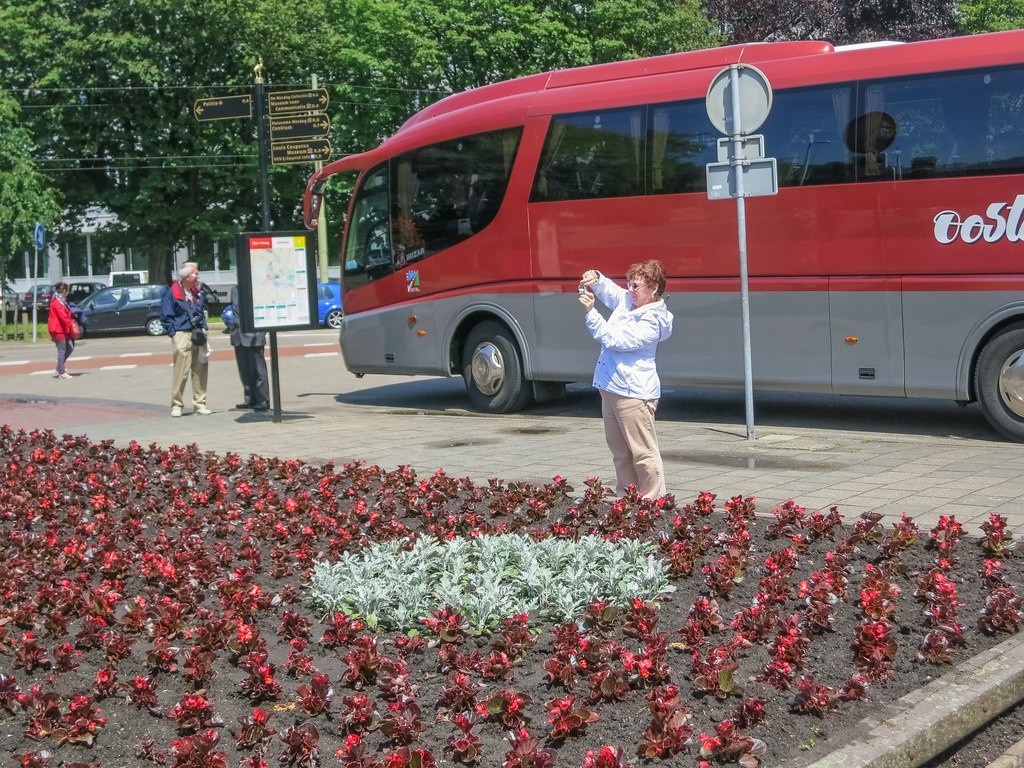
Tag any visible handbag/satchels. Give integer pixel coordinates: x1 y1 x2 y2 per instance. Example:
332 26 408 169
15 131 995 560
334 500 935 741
190 328 208 346
69 318 80 340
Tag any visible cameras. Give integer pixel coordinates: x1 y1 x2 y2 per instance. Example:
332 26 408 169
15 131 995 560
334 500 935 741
579 285 587 295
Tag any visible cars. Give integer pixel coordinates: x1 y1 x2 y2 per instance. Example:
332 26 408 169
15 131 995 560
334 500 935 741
69 285 170 341
223 282 345 329
25 284 54 304
0 285 20 311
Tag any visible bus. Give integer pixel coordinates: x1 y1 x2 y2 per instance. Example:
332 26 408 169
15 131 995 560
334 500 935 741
303 27 1024 446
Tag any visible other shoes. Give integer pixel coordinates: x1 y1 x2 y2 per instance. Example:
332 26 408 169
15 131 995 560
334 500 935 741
254 404 271 411
236 403 253 410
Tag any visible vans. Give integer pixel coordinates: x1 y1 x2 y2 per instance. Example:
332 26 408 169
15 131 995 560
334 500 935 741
70 282 107 306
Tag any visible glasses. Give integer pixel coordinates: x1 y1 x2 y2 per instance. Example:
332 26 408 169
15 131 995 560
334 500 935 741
627 281 643 290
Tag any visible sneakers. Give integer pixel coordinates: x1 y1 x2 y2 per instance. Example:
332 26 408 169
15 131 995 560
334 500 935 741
58 373 73 379
54 369 67 376
171 406 182 417
192 408 212 415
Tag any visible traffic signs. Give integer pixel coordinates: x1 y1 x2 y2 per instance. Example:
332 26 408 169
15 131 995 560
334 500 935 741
270 113 330 140
272 137 332 164
268 88 329 116
193 95 251 121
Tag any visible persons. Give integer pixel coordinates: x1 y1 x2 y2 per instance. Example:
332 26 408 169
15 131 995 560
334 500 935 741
160 262 211 417
578 260 674 501
48 283 78 379
230 284 270 411
